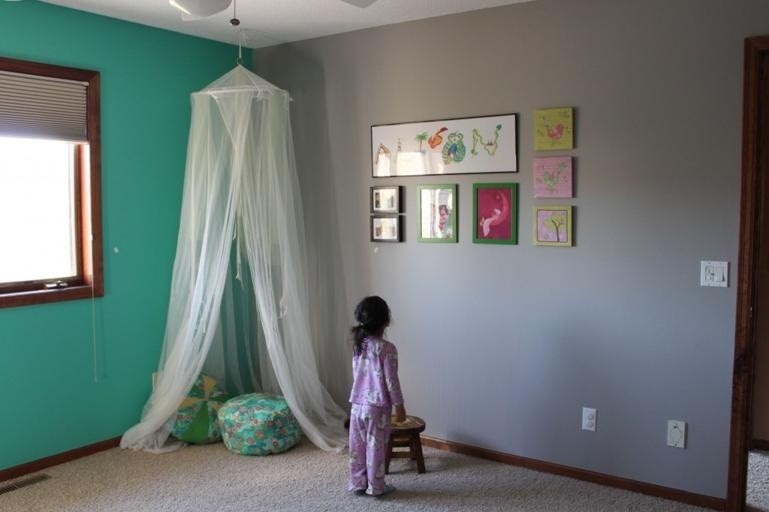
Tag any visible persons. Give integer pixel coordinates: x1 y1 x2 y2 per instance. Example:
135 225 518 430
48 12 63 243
346 295 406 495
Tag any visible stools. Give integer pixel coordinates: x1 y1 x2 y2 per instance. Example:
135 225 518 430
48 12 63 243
384 414 426 475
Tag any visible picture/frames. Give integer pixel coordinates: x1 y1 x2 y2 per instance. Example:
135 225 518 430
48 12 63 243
416 183 458 243
370 112 519 178
369 186 403 214
471 182 518 245
531 205 573 248
369 215 403 242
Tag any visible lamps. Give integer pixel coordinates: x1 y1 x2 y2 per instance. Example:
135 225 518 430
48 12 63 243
167 0 232 20
